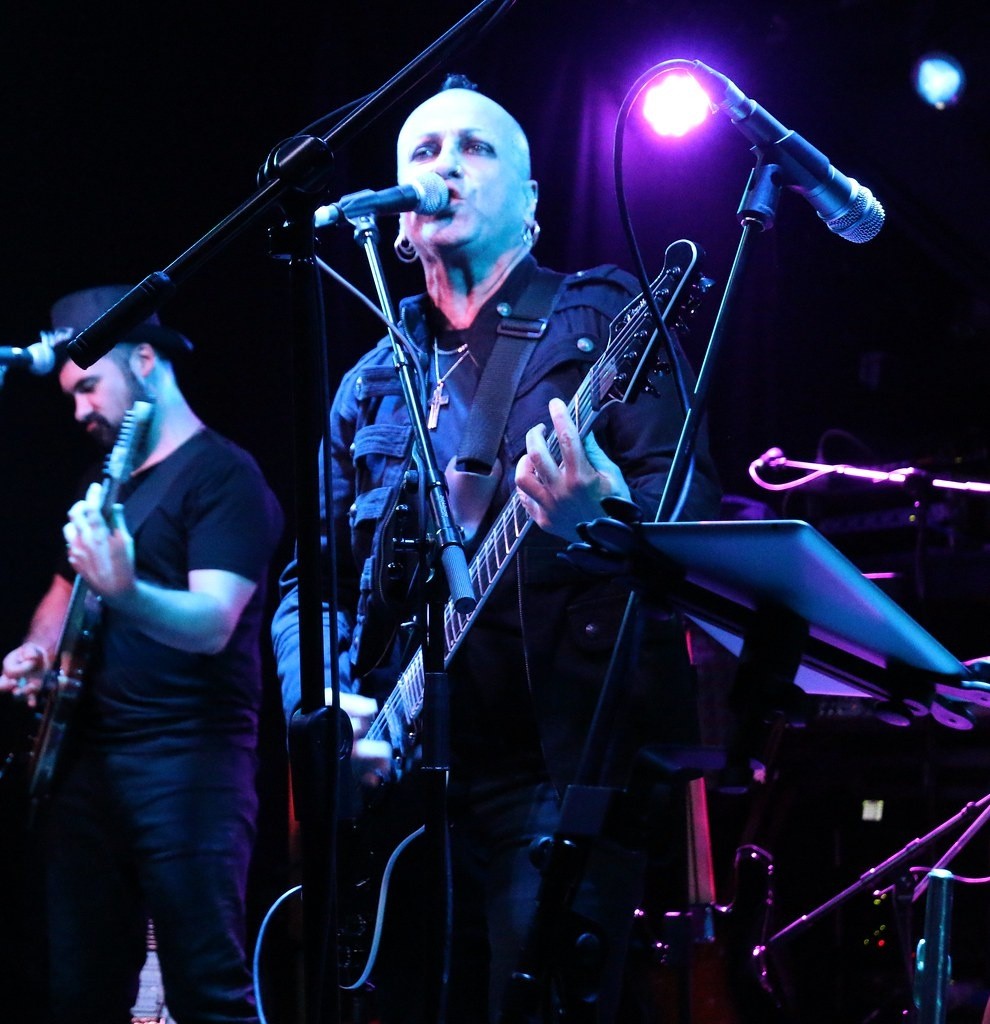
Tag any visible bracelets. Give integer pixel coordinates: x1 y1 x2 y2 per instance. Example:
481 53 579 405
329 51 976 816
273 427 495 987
26 640 50 674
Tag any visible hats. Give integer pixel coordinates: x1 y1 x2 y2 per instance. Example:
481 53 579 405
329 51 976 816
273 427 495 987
48 284 194 369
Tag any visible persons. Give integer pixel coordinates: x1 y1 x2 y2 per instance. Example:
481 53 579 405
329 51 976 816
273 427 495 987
0 284 294 1024
269 75 721 1024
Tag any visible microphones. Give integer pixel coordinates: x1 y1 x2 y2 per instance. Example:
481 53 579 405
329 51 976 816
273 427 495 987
755 864 787 991
315 172 449 228
750 448 784 471
692 60 886 243
0 343 57 377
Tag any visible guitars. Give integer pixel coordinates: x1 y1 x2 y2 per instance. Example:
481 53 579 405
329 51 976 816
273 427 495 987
339 230 717 841
22 394 153 801
624 630 783 1024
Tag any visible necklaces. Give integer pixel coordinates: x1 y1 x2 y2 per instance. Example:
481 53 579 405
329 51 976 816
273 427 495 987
427 336 469 430
